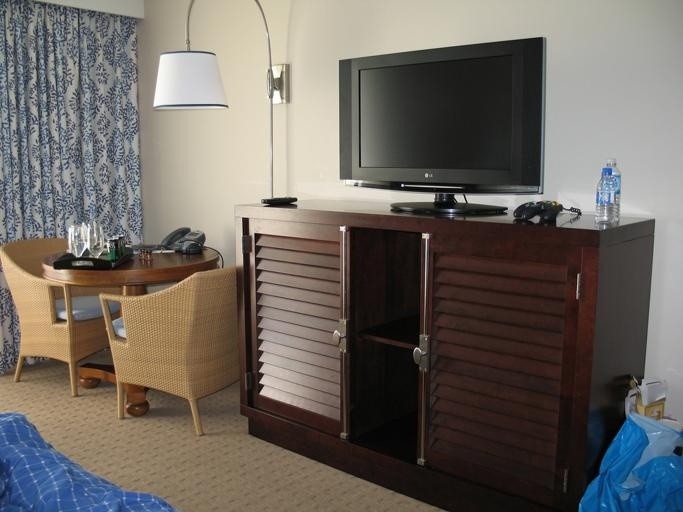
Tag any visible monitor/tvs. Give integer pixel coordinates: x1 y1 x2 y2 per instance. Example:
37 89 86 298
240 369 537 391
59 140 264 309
339 36 547 216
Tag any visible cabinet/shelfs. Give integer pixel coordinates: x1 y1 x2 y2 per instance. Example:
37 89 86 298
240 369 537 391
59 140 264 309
232 197 655 512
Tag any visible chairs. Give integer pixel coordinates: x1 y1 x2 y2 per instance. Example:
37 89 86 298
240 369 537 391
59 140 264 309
99 264 241 436
1 236 121 396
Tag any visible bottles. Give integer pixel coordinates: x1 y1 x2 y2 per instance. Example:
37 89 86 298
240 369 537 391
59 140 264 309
595 159 621 224
139 248 153 261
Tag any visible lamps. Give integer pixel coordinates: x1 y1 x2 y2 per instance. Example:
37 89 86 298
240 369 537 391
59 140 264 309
151 1 290 198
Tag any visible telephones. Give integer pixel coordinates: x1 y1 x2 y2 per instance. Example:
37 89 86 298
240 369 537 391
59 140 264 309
161 227 205 252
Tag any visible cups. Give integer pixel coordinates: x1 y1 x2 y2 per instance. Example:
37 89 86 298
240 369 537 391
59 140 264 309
69 221 105 257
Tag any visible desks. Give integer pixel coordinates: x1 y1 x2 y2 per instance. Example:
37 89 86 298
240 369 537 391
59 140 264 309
41 244 220 415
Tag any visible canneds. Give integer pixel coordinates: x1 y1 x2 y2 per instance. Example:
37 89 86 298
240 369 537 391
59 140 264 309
106 234 125 264
